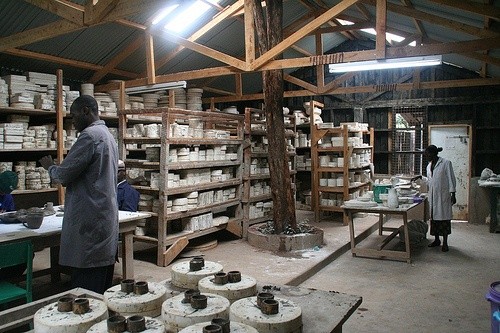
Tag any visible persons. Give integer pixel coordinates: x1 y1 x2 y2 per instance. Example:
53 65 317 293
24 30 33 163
1 171 35 284
410 145 456 252
40 94 120 295
117 160 140 212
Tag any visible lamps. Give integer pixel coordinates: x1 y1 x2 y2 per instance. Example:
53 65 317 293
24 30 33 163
329 54 443 74
125 80 187 94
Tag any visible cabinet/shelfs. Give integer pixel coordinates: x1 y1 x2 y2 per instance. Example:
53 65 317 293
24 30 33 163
0 69 375 266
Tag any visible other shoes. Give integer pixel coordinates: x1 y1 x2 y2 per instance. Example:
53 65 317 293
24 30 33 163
428 240 441 247
442 245 448 252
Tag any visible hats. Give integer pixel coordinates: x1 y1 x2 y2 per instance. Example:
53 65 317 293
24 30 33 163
118 160 126 170
0 171 18 194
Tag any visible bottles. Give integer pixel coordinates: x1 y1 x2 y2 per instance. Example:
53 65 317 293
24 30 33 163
387 188 399 209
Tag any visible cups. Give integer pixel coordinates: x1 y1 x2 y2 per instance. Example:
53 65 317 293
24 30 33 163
257 292 279 315
190 257 204 272
184 290 207 309
44 202 53 209
214 271 241 284
202 317 231 333
23 212 43 229
107 314 145 333
120 278 148 295
57 296 89 314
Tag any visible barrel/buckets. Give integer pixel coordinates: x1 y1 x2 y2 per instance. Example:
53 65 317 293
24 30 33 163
373 184 393 203
485 281 500 333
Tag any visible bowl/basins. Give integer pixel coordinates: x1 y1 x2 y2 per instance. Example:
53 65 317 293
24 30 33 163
320 136 370 207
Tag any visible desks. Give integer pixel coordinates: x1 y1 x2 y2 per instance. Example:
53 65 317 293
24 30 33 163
340 193 428 265
477 183 500 233
24 278 362 333
0 204 151 280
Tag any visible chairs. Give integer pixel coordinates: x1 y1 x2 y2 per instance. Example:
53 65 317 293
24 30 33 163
0 240 34 330
